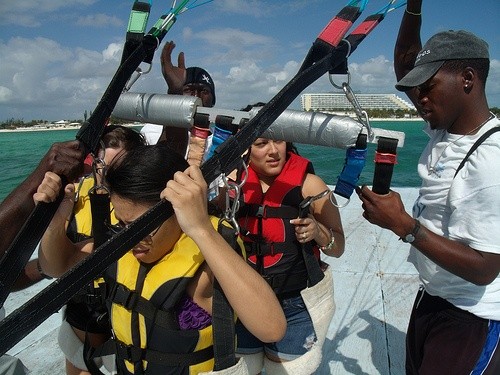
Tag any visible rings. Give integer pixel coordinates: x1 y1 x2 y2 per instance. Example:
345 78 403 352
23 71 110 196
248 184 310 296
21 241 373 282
302 233 306 240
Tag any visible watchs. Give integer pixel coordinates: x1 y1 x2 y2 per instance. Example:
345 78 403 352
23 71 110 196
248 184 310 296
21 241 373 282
399 219 421 244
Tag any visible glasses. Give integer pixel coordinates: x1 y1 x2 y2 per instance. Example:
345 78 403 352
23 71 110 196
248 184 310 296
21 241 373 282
95 167 109 178
103 206 162 242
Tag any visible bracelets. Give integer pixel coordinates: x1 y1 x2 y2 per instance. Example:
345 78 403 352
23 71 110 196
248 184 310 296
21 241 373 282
321 228 336 251
405 10 421 15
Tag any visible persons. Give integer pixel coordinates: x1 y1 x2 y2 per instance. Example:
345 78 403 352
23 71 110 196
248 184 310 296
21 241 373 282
354 0 500 375
32 144 288 375
0 140 84 259
38 124 147 375
206 103 345 375
145 40 216 170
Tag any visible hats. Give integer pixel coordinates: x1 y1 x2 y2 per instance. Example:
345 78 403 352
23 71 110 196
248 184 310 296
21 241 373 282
394 29 489 91
184 67 216 104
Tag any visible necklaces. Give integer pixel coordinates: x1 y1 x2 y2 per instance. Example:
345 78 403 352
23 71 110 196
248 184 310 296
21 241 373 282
427 114 499 175
